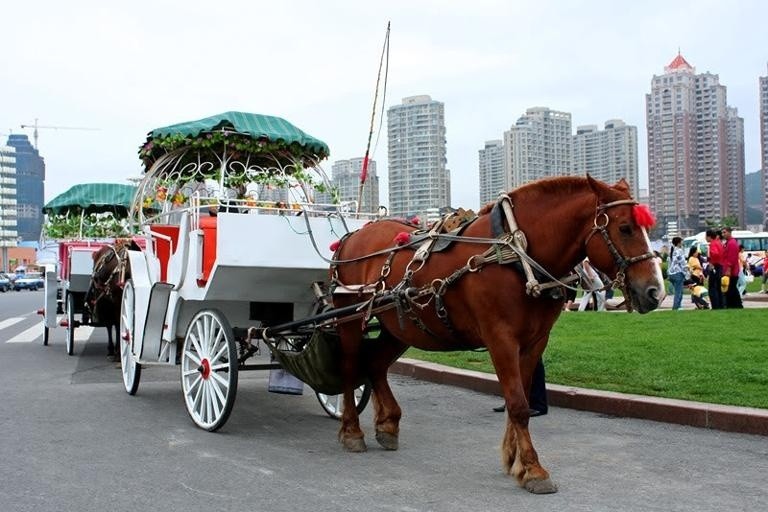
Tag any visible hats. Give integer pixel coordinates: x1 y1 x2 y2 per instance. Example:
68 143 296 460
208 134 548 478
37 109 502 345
720 276 730 293
688 279 697 286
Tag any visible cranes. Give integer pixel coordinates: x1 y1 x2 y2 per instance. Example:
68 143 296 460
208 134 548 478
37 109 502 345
21 118 101 150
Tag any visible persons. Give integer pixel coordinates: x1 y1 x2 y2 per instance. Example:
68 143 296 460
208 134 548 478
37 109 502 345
565 257 614 311
653 227 752 310
758 250 768 293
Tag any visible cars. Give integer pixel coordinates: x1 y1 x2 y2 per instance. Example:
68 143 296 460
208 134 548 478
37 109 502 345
0 266 44 292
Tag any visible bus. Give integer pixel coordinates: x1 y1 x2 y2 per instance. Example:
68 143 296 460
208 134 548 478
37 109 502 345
680 230 768 269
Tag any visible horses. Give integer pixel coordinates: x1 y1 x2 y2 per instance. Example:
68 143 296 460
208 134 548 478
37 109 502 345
327 171 669 495
91 238 143 361
